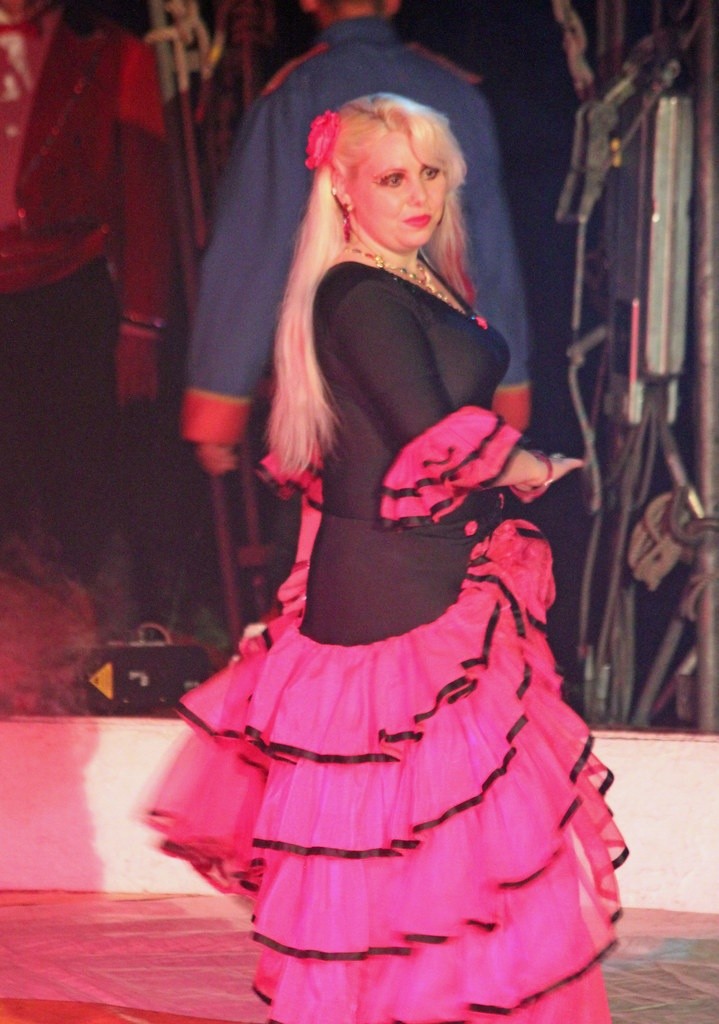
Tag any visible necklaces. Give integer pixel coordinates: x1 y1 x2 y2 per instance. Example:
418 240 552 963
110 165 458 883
343 245 489 331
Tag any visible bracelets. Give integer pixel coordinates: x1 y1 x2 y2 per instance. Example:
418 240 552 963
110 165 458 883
508 451 553 503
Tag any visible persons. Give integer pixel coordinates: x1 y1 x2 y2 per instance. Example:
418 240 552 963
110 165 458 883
132 92 631 1020
181 0 537 584
0 1 172 628
